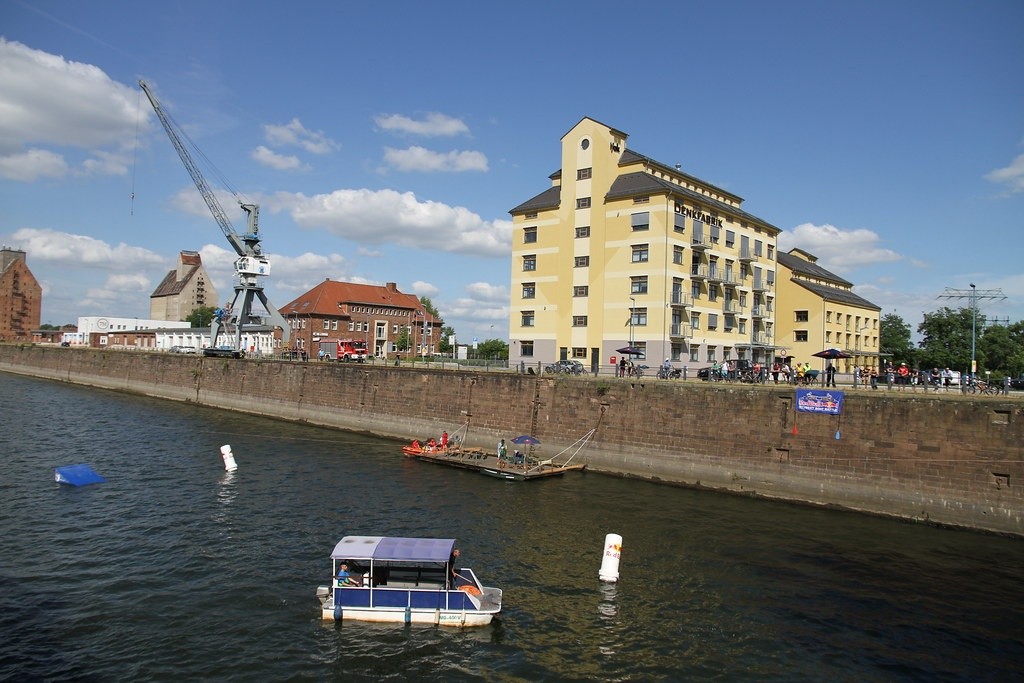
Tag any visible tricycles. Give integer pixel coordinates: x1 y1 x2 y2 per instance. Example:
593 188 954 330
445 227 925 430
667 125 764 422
795 370 819 386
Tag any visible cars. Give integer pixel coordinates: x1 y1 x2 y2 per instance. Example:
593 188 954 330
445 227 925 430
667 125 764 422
170 346 196 354
61 342 70 347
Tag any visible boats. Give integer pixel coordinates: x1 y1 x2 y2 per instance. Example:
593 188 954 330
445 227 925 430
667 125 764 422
316 536 502 626
402 447 456 456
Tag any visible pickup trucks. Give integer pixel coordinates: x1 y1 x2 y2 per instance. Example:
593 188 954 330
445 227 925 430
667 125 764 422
697 359 769 382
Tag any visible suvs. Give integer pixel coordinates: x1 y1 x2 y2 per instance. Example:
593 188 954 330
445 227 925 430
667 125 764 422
922 369 951 383
545 360 583 372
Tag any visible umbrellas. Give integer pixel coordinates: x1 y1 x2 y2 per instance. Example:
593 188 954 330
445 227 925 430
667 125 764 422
616 346 644 361
510 435 541 453
811 349 853 386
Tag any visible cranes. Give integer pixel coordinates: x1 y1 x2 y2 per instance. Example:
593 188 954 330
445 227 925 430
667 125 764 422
138 80 270 285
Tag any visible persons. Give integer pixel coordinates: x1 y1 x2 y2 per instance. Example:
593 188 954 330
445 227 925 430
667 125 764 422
497 438 508 469
284 346 325 362
337 560 363 587
619 357 634 378
393 354 401 365
412 431 448 455
664 358 671 379
826 363 836 388
853 362 1012 394
443 548 461 590
711 358 812 384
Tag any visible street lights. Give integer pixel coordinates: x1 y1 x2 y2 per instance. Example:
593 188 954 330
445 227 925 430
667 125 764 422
970 283 976 384
421 304 426 354
293 311 299 346
197 314 202 349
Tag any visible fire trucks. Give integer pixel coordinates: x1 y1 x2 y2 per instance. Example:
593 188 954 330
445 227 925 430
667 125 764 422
319 339 368 362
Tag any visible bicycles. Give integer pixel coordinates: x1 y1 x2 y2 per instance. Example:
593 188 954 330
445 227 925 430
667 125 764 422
629 365 644 376
656 367 681 380
968 378 1003 396
547 363 588 376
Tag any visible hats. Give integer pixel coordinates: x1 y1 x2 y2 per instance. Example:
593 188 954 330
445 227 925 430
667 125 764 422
901 363 905 365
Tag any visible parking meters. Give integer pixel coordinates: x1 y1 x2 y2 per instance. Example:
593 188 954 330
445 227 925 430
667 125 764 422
630 296 635 363
